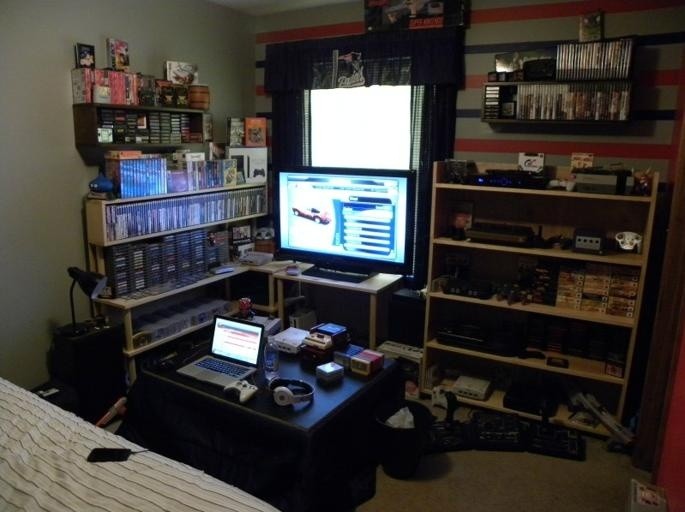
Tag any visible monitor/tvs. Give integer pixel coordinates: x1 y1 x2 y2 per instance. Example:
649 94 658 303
270 164 417 283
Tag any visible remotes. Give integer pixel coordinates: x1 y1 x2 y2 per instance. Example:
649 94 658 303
209 265 233 275
286 264 299 276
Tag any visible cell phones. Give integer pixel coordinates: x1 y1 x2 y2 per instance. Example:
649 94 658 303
87 448 132 462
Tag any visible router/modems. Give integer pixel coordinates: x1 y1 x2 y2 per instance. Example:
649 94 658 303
239 251 274 267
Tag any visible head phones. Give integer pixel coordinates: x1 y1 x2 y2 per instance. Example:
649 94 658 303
267 375 315 407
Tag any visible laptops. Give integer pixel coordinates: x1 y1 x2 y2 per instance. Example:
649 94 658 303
175 314 265 387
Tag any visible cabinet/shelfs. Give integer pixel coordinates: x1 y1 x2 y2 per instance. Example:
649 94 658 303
424 159 659 433
277 264 403 348
483 77 651 137
73 101 285 390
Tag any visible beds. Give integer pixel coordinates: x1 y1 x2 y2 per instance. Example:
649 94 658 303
1 374 289 512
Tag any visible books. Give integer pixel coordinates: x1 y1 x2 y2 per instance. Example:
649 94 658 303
164 61 199 84
74 42 96 69
578 10 603 42
526 258 641 320
226 116 273 148
518 152 546 176
105 185 268 296
104 148 244 197
570 152 598 170
517 40 631 119
73 67 210 147
105 37 131 71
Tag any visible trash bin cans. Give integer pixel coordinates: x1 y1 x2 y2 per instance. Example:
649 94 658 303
376 400 438 480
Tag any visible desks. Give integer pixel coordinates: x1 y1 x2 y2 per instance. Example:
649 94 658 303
113 357 406 512
141 325 415 440
